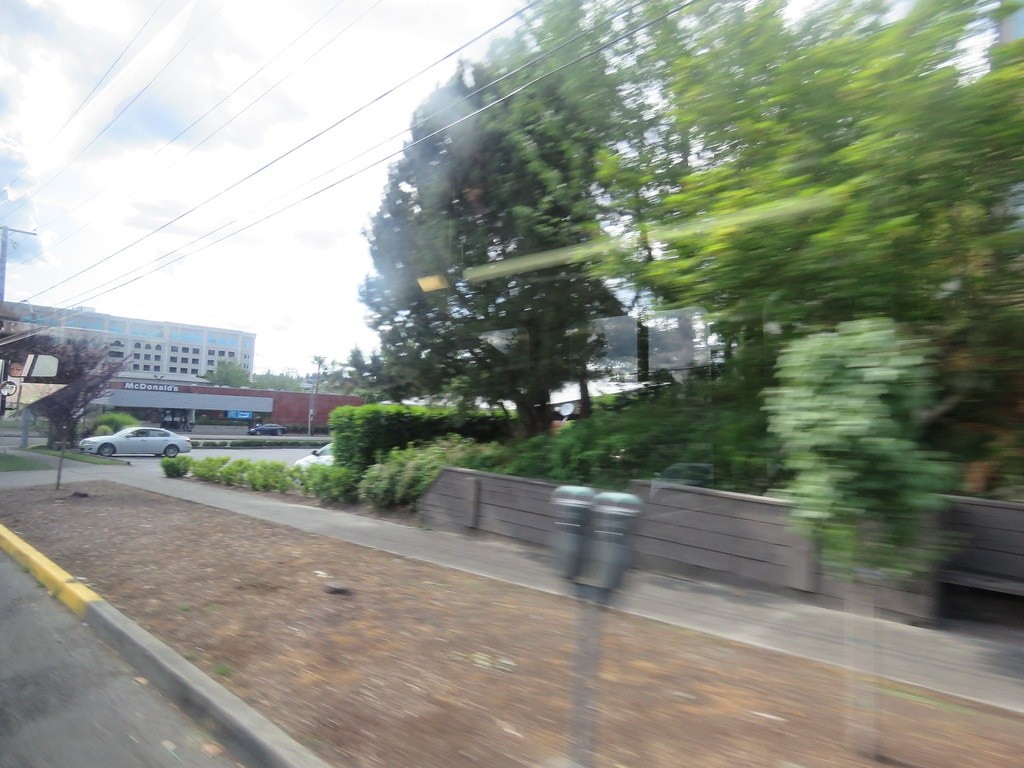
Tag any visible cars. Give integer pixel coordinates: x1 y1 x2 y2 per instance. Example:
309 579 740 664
247 424 288 436
293 443 335 470
79 427 192 458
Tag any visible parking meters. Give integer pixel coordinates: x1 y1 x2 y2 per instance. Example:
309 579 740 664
548 484 642 768
